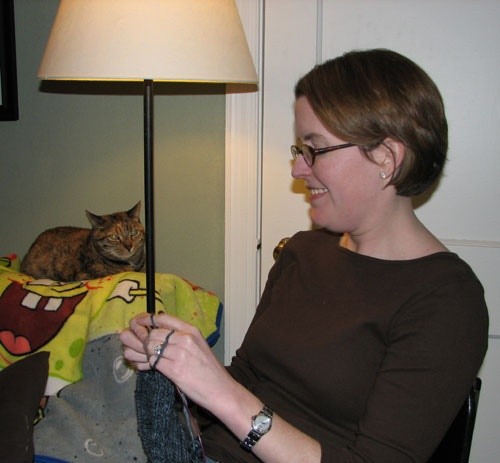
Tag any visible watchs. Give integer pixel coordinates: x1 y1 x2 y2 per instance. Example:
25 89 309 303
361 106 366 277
241 404 274 450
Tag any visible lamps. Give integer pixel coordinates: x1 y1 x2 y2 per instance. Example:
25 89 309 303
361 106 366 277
38 0 260 313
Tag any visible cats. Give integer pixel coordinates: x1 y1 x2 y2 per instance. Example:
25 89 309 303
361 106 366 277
19 201 147 283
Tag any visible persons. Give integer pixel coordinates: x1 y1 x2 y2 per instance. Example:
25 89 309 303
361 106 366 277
122 48 489 463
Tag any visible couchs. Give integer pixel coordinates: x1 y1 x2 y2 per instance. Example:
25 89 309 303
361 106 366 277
0 256 226 463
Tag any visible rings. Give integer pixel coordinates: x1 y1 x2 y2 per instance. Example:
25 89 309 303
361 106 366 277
154 343 163 356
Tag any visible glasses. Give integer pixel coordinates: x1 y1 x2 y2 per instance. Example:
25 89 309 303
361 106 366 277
291 143 355 167
